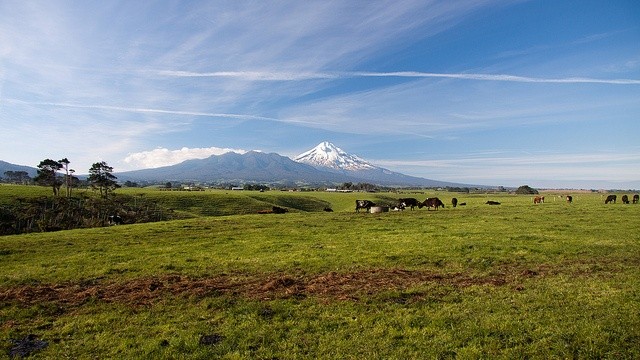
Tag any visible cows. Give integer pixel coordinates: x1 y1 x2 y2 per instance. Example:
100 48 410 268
540 195 544 202
438 198 444 208
631 193 639 203
485 200 500 204
256 209 267 214
390 204 404 211
459 201 466 204
272 205 289 214
604 194 616 204
565 195 572 203
104 213 122 225
267 209 273 212
418 196 439 210
398 197 421 211
621 193 629 203
451 196 457 207
355 198 376 212
322 205 333 212
533 194 540 203
